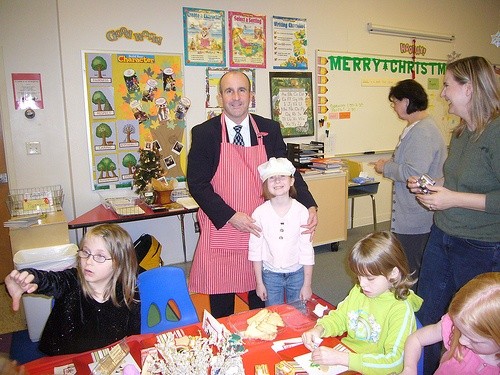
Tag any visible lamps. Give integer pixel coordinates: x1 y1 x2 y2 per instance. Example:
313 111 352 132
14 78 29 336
367 23 456 42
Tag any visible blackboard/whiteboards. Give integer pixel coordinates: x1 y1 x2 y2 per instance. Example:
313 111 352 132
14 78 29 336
315 49 451 159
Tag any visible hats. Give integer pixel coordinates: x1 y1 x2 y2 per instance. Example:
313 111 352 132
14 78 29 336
258 156 296 182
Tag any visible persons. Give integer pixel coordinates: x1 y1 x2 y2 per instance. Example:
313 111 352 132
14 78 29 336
249 157 315 307
413 55 500 375
375 80 443 294
187 71 317 320
303 230 424 375
398 273 500 375
4 225 141 355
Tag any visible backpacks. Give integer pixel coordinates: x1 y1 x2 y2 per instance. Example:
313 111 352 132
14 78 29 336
131 234 164 274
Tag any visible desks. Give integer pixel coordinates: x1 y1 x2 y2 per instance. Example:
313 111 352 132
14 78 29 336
15 305 362 375
68 197 200 263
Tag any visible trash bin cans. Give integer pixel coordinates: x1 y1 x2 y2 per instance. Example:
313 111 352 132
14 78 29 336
12 243 79 344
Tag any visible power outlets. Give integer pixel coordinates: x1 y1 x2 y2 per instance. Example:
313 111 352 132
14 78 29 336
193 222 200 232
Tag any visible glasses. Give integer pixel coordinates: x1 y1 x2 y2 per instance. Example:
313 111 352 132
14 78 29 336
77 249 112 264
391 99 399 107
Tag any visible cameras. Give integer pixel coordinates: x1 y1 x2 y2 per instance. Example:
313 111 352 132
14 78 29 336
416 173 435 194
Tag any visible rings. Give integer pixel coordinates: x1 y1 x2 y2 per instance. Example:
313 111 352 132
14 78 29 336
430 206 433 211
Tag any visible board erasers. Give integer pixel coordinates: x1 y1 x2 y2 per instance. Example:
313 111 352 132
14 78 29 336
363 151 375 154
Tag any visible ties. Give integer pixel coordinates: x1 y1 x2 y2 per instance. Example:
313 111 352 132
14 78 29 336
233 125 245 147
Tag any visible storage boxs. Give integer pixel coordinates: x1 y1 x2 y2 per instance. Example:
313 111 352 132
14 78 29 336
349 181 379 196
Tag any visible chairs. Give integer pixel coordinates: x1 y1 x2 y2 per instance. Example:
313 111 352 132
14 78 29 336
342 160 380 231
136 265 200 334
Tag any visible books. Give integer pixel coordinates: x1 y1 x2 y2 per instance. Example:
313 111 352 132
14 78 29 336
299 157 345 175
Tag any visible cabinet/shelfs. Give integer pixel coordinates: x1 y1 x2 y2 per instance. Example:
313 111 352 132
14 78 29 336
301 170 348 252
